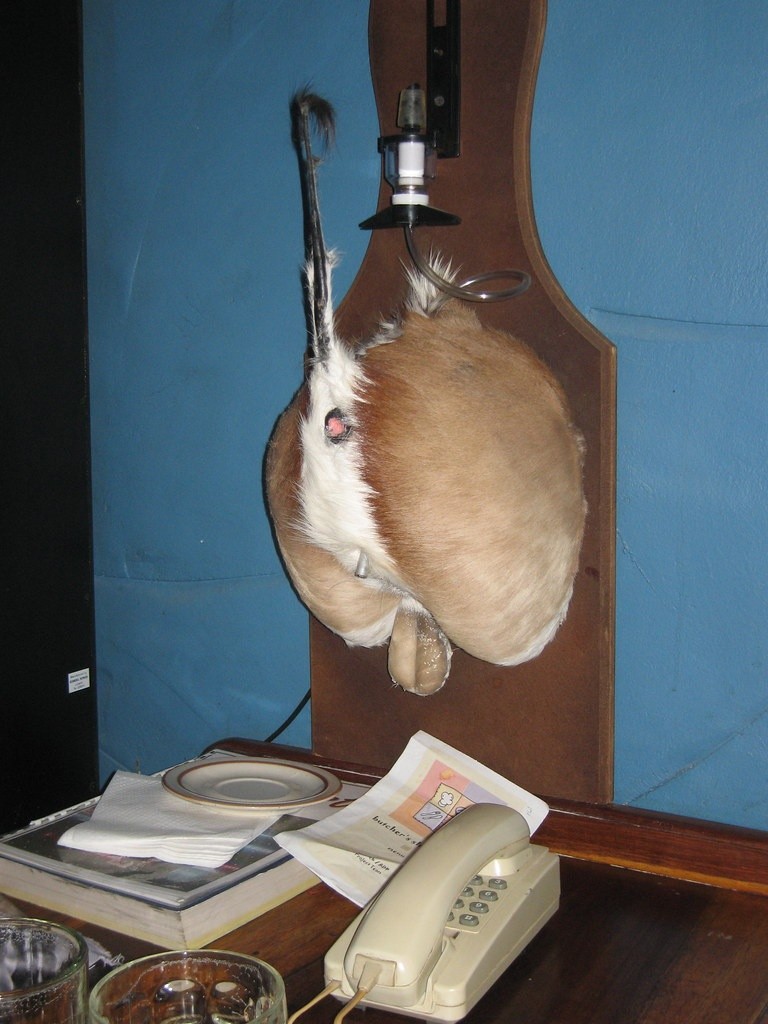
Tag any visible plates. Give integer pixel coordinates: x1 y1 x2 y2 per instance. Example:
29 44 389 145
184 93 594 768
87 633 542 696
161 757 344 816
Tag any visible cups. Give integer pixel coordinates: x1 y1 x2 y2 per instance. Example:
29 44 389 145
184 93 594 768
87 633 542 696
0 917 90 1024
88 949 288 1024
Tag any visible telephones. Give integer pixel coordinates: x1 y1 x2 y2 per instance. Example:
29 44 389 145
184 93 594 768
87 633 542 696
323 802 563 1024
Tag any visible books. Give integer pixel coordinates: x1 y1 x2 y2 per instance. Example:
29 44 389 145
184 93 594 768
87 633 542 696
0 746 381 954
